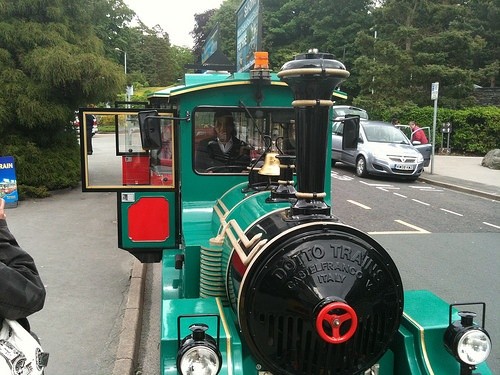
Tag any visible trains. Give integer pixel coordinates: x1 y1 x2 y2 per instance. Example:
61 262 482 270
78 50 494 375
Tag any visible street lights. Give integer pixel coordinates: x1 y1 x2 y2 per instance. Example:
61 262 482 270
115 47 127 152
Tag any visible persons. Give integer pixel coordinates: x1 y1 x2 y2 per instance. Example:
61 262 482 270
86 114 93 155
0 198 46 346
194 111 252 173
392 118 405 133
409 121 429 144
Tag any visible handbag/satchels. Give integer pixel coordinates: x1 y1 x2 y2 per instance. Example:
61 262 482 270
0 319 50 375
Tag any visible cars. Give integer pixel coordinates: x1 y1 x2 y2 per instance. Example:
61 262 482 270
70 110 98 138
331 105 432 182
